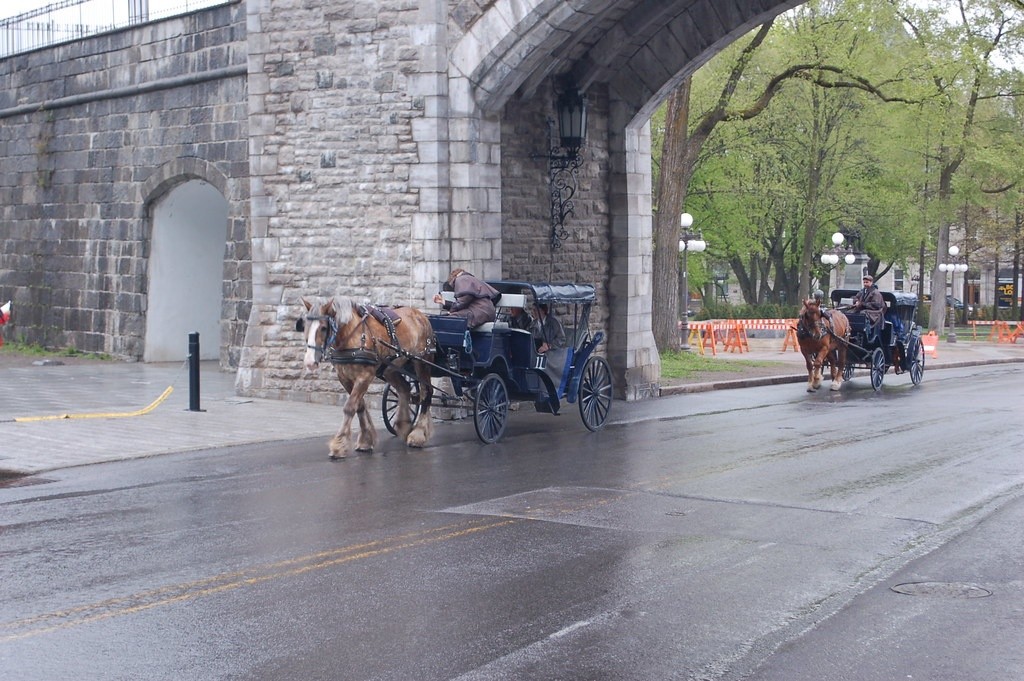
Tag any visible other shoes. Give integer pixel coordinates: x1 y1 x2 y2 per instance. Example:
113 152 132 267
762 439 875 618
471 349 481 360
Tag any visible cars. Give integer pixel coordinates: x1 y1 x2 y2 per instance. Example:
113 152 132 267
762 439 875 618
922 294 975 322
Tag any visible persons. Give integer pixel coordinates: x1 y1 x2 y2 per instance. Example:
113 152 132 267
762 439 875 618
432 269 502 361
883 303 906 343
846 275 885 341
503 303 566 354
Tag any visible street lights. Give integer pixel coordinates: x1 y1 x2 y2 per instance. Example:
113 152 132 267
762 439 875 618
678 213 705 349
939 246 968 343
821 233 855 306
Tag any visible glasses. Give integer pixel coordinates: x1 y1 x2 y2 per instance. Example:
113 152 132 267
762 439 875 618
864 282 869 284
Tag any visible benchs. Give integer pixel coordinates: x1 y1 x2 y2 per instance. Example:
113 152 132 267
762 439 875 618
563 327 590 368
469 322 509 362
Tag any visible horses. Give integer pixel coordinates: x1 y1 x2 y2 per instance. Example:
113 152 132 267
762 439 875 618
300 294 435 460
796 298 852 393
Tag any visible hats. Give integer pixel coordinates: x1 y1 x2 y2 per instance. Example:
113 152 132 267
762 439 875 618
863 275 873 281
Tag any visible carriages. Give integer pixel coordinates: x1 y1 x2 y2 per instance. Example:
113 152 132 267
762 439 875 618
796 288 925 392
296 281 614 458
